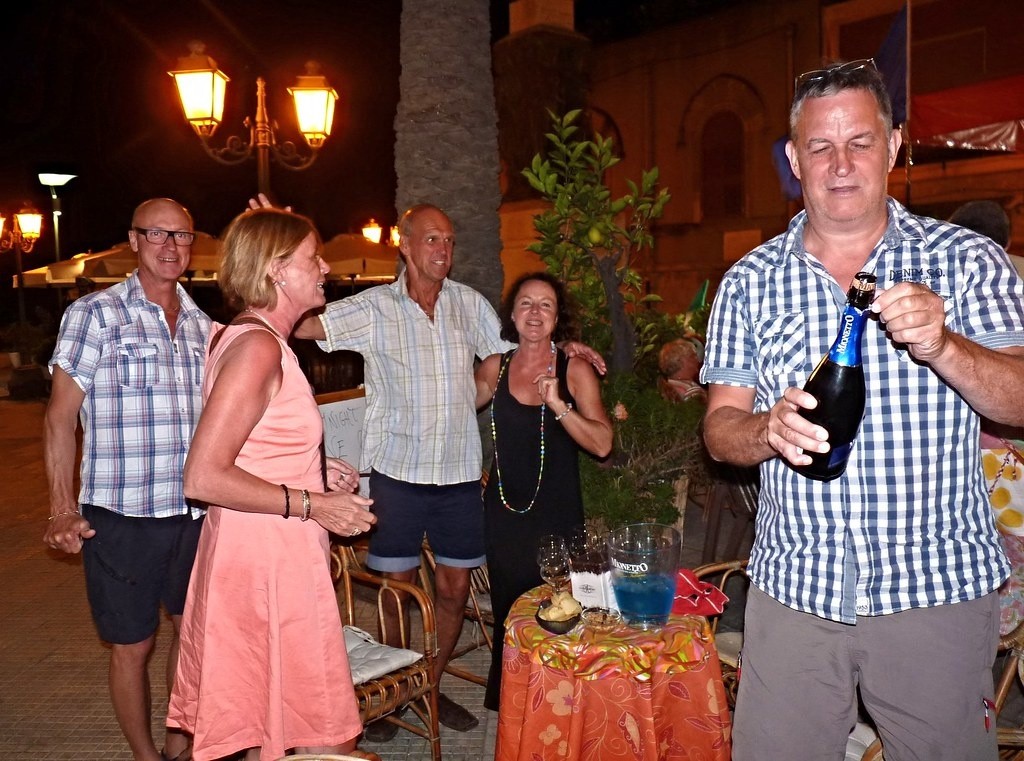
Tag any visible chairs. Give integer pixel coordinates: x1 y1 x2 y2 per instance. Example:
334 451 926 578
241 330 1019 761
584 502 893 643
325 518 522 761
693 559 748 703
985 622 1024 748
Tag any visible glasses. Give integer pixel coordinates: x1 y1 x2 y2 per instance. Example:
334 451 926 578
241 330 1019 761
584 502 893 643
794 56 882 100
133 226 198 247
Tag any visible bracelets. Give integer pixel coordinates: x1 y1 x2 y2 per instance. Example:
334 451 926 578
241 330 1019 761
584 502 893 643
48 510 81 520
555 401 573 422
298 488 311 521
281 484 290 519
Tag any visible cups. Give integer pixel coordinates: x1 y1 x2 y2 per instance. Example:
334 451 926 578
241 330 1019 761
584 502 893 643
605 521 681 629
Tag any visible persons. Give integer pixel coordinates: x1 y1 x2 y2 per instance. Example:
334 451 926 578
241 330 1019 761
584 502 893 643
475 274 614 713
658 334 708 404
0 250 24 368
698 63 1024 761
43 199 213 760
246 191 606 744
167 207 378 761
33 275 96 368
945 200 1011 252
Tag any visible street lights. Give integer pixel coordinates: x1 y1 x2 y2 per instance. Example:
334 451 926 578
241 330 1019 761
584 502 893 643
37 169 80 315
166 40 340 199
0 200 50 400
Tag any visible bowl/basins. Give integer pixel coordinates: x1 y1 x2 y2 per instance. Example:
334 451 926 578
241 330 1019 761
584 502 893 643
535 608 581 635
581 608 622 633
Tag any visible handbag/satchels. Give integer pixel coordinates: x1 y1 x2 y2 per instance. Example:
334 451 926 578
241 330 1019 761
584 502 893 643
670 567 728 618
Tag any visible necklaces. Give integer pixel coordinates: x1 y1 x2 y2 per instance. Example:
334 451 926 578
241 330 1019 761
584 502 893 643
491 340 556 512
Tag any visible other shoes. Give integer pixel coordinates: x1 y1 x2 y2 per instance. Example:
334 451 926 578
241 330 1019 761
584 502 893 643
420 690 480 733
364 708 407 740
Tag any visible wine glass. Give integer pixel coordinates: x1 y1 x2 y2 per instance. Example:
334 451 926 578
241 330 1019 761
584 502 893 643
538 523 607 603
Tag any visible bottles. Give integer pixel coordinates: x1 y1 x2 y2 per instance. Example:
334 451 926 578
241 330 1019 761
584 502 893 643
782 272 877 481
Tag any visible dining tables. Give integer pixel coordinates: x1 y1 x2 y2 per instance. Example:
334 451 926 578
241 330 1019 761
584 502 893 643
494 580 731 760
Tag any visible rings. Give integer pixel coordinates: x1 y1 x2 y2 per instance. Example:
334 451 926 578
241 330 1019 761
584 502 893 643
350 527 362 536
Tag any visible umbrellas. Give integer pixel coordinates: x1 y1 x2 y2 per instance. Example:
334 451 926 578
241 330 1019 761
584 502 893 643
23 231 405 299
